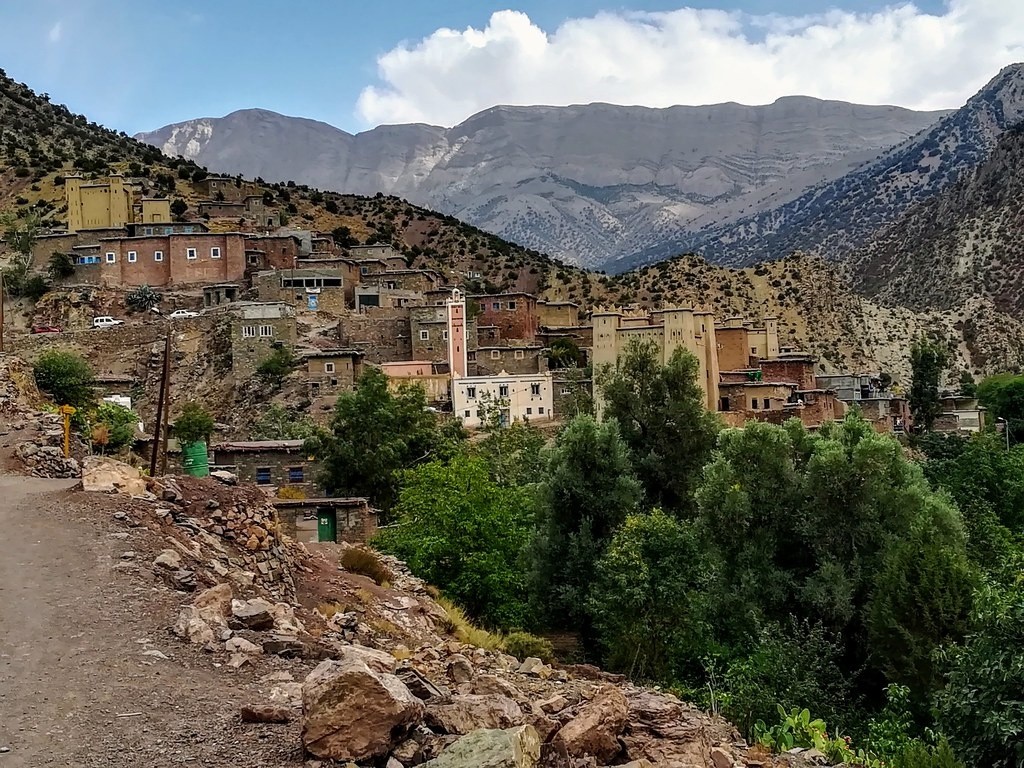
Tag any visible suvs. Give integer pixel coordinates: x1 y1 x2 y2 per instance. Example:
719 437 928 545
93 317 125 328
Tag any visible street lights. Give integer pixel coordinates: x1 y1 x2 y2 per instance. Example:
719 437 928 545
998 417 1009 452
291 256 298 304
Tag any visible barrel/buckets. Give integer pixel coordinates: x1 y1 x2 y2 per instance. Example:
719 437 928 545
181 441 211 478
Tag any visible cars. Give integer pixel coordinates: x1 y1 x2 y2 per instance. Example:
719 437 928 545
30 325 62 334
170 309 200 319
